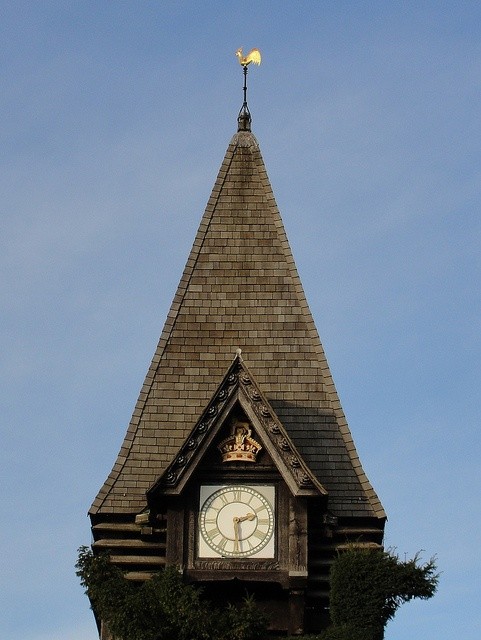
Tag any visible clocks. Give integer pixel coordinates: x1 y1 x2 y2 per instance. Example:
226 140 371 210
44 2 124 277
198 485 276 558
